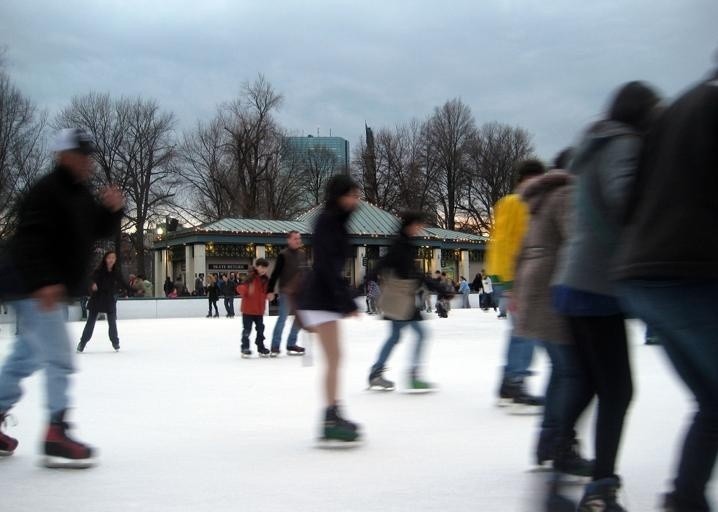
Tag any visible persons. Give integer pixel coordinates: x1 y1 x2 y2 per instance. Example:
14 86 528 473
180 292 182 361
235 229 306 358
298 174 358 450
358 212 485 394
194 273 239 319
164 277 186 298
1 128 145 469
483 52 717 511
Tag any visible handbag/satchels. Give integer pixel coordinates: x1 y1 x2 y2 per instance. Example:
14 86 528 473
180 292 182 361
377 274 418 321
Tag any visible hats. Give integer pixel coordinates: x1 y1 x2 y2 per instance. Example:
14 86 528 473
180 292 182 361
48 129 104 156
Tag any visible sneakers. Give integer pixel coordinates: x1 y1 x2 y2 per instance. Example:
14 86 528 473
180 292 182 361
98 315 105 321
533 423 711 510
112 341 120 350
205 313 234 317
406 378 430 389
321 418 359 442
1 429 18 456
498 381 513 400
369 375 394 388
42 421 92 460
77 340 86 350
240 344 304 352
80 316 88 321
482 305 506 314
506 387 544 407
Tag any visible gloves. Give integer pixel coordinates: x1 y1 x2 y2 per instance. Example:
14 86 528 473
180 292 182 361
439 287 455 301
